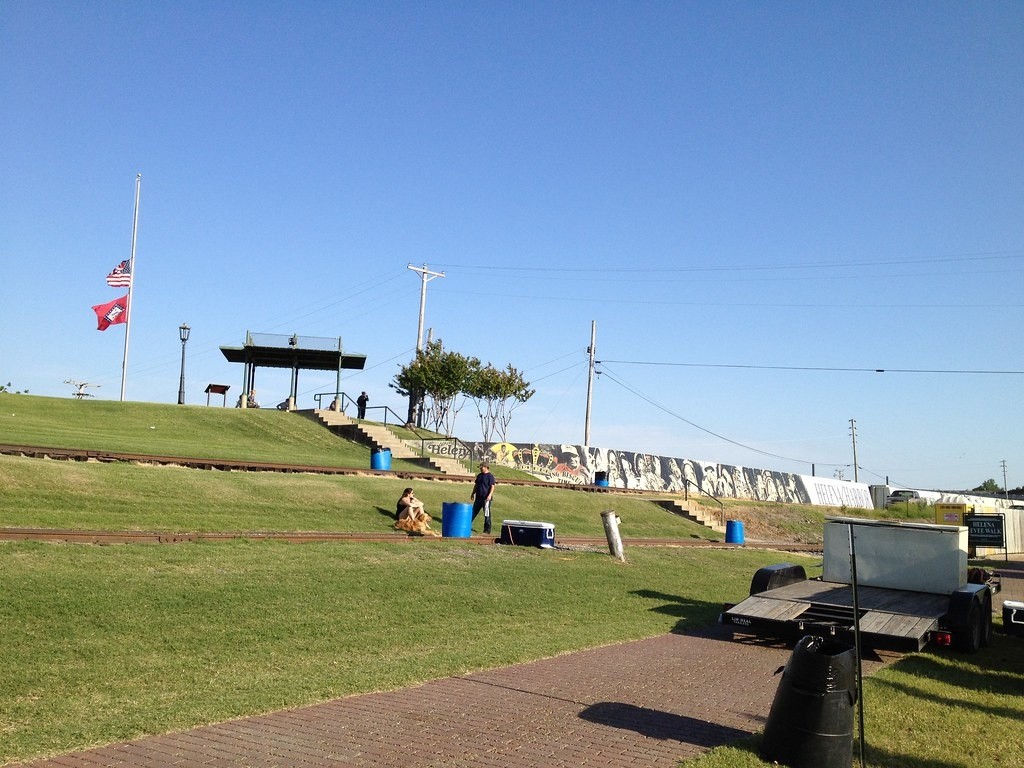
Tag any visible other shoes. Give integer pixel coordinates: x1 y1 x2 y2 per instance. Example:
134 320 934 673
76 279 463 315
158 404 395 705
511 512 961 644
481 531 491 535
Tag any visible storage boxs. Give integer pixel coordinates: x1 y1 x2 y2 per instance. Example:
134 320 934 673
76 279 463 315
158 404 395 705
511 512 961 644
500 520 555 549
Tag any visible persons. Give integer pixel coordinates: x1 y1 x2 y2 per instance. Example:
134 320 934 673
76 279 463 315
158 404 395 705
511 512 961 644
329 396 338 411
394 488 431 529
470 461 496 535
496 445 511 463
552 444 804 509
276 398 291 411
235 389 260 409
355 391 370 419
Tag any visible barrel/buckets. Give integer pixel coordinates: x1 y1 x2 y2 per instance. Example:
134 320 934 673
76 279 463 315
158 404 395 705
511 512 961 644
442 502 473 538
758 637 855 768
371 448 391 470
726 521 744 544
595 472 609 487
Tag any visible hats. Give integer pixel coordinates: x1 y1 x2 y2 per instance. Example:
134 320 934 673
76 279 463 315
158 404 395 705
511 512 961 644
476 462 489 468
362 392 366 394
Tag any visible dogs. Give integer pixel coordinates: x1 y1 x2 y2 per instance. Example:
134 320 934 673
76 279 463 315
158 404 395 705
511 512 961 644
393 512 441 538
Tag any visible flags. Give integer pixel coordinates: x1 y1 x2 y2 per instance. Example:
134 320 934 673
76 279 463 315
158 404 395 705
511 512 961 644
105 260 131 288
93 294 126 330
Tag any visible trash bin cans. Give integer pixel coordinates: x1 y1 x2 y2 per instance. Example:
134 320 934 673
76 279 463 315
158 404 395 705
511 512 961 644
441 502 473 538
758 634 855 768
370 447 392 470
595 472 609 486
726 520 744 543
